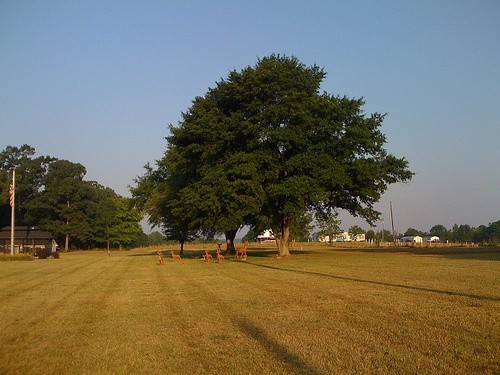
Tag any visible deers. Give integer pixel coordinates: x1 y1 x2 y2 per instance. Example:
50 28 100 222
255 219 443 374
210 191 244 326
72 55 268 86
170 250 182 261
204 250 212 262
216 249 224 263
235 242 248 259
156 250 164 264
